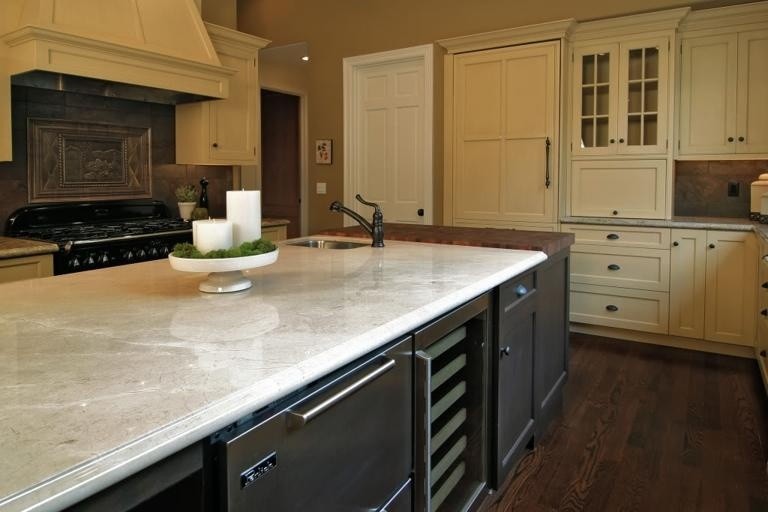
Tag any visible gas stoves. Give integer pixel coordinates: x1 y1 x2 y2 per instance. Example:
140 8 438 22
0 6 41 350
23 217 190 273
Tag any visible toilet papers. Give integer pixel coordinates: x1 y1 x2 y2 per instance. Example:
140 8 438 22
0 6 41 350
192 220 233 251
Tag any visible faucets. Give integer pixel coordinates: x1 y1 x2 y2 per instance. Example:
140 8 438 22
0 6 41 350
328 194 385 248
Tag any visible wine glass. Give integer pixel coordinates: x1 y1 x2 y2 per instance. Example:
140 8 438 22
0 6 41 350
168 246 280 294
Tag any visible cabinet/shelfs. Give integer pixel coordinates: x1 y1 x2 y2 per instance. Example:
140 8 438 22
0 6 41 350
675 0 767 160
560 4 692 222
670 228 761 360
497 272 537 489
197 332 414 512
434 15 576 233
174 20 272 167
561 224 670 347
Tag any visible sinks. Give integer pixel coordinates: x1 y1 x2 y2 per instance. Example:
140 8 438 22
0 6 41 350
285 238 370 250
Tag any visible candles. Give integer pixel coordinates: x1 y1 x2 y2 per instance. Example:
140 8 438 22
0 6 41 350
191 215 233 254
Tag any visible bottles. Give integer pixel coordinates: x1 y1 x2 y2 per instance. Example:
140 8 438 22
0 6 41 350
200 179 210 207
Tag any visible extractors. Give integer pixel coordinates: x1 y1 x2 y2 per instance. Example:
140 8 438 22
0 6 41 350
11 2 233 106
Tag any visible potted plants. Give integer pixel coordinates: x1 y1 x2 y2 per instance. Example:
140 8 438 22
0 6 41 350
172 181 198 220
